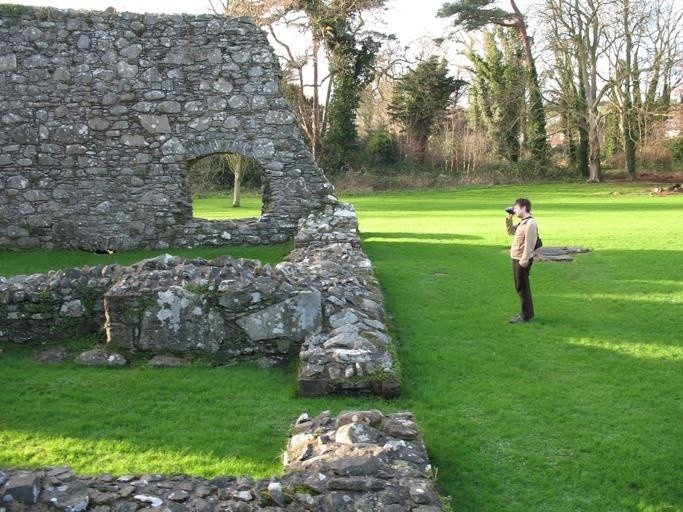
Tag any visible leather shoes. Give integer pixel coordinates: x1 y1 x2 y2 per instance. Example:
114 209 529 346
511 314 533 322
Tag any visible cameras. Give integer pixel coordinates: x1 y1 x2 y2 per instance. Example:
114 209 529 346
505 206 514 214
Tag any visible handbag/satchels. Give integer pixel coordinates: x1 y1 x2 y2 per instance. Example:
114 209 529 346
534 237 542 250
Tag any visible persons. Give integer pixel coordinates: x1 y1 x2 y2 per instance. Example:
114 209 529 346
506 199 538 324
